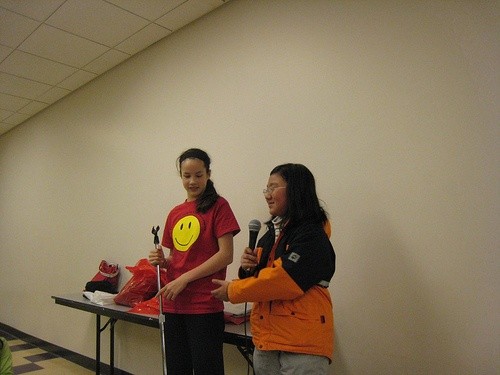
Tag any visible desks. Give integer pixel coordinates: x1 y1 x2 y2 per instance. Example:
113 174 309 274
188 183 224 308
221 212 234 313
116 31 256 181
51 293 256 375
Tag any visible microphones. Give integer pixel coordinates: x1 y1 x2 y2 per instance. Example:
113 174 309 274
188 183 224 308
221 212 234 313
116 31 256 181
246 219 261 273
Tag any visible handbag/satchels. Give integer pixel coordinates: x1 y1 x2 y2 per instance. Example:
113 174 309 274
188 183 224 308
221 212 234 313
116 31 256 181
114 258 159 309
85 260 120 294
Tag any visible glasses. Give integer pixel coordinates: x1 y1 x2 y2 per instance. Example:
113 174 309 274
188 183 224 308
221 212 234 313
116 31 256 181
263 186 287 194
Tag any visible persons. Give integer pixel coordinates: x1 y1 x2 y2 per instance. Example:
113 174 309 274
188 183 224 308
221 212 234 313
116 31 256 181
210 163 336 375
149 148 242 375
